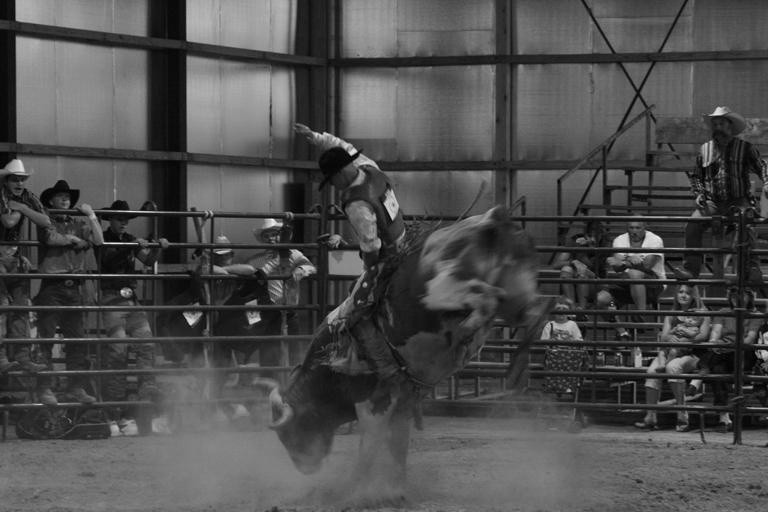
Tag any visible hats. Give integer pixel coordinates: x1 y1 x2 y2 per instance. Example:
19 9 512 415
98 200 138 221
0 159 33 178
318 146 362 192
702 107 746 135
253 218 293 244
204 235 233 254
40 181 79 209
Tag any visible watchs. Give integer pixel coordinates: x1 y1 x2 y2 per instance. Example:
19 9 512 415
623 253 634 261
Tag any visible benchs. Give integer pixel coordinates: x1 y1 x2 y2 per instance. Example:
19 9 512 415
465 117 768 416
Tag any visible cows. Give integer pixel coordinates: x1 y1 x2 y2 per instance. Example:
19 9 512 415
248 201 548 501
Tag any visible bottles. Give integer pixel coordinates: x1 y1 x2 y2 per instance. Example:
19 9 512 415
634 346 643 368
615 353 623 366
597 353 605 367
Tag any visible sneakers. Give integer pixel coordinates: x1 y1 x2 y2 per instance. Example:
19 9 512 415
684 384 705 400
2 354 20 372
38 387 59 408
67 389 97 405
21 361 50 371
671 265 697 285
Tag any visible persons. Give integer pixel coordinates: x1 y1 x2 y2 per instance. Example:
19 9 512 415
674 105 768 282
294 123 407 401
686 284 765 432
655 310 704 372
35 180 104 405
156 218 317 429
92 200 169 402
598 214 667 332
541 300 583 395
554 222 607 311
634 284 711 432
2 159 51 372
